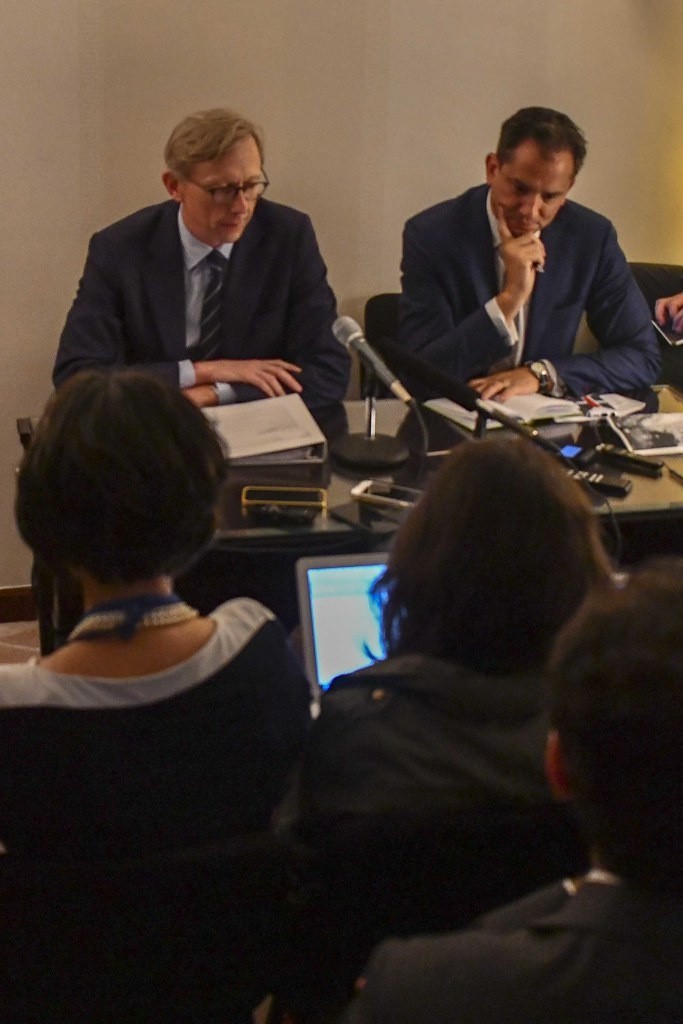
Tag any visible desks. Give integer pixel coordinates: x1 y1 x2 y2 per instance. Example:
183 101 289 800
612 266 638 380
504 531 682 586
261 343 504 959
60 399 683 654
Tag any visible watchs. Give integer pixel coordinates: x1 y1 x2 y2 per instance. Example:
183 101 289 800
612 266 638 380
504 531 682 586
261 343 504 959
524 360 547 394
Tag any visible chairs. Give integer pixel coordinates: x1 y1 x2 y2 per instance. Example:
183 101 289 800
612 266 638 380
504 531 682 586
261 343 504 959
626 260 683 390
362 293 415 394
297 801 586 1024
0 830 290 1024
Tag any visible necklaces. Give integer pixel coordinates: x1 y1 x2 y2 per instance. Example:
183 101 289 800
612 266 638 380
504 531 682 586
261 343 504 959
68 597 197 642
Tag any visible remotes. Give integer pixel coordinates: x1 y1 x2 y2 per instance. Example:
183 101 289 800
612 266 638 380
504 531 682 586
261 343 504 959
561 470 633 496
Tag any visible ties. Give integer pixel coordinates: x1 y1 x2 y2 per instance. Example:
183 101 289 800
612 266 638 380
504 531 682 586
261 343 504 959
498 270 523 373
200 249 228 360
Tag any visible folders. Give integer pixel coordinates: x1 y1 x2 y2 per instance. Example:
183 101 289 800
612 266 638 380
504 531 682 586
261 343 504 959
198 393 327 465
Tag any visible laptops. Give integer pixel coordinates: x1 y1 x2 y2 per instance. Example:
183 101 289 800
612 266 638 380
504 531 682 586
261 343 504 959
650 320 683 346
295 552 393 720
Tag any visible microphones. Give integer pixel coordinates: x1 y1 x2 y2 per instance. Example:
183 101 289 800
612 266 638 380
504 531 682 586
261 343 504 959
332 316 415 408
374 340 562 456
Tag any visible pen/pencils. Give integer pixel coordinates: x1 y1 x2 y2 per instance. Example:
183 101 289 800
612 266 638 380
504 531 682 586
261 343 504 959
532 261 545 273
364 369 377 439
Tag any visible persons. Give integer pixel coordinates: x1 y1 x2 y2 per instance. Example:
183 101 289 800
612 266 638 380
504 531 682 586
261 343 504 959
654 292 683 333
52 110 352 409
1 373 313 1024
398 106 659 408
250 437 683 1024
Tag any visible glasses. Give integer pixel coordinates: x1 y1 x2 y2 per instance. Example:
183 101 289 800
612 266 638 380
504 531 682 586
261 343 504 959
183 168 270 203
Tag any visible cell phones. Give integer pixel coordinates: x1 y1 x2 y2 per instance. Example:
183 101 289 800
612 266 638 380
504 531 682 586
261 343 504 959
351 479 423 511
242 486 327 513
